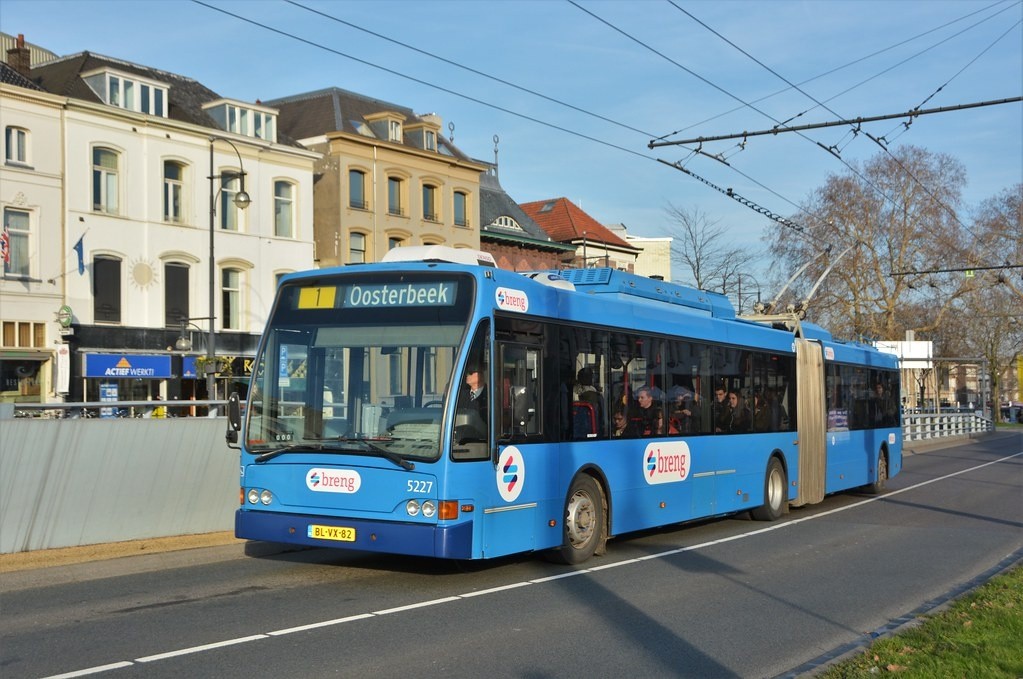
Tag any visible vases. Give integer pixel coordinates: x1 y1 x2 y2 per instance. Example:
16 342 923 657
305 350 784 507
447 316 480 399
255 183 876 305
205 361 224 373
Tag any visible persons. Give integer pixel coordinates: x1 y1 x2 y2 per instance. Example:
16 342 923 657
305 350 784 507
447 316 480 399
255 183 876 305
452 359 496 444
828 380 901 434
572 368 789 437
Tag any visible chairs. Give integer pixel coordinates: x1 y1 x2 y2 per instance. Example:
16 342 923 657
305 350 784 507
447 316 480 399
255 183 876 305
572 402 681 438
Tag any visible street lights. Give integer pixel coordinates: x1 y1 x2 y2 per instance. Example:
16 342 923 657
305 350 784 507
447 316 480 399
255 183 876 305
736 272 769 316
175 135 254 418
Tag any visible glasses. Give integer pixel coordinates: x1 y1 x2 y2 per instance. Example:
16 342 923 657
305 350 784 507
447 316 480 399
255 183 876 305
464 370 478 375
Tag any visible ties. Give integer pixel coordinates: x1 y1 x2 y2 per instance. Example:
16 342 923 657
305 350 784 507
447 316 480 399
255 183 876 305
470 392 475 401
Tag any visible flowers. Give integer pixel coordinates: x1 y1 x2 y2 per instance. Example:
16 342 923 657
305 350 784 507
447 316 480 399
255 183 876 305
192 355 232 379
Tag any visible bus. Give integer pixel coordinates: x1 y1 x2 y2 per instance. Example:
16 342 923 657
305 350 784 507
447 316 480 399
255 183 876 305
225 241 903 568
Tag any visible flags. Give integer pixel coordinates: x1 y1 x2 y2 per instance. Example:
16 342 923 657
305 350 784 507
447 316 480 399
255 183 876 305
0 224 11 267
73 236 85 275
